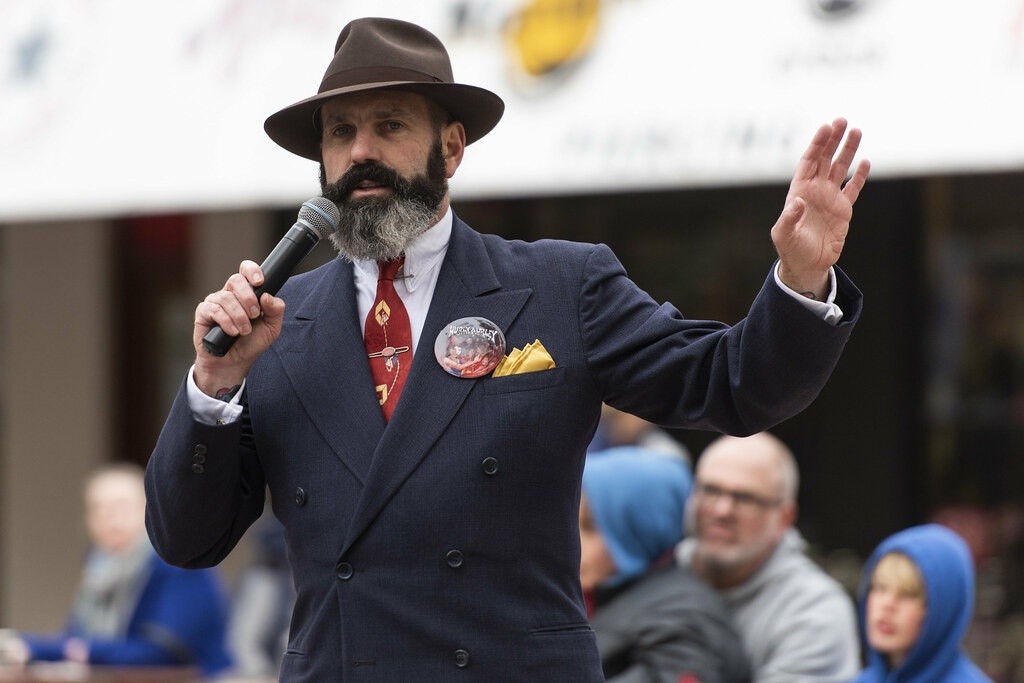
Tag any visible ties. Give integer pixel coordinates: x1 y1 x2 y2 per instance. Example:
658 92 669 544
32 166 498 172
363 253 413 423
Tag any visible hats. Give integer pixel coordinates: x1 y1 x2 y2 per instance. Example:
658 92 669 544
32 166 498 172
263 17 505 162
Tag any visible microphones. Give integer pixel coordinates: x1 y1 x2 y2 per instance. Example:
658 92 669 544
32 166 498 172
202 197 340 356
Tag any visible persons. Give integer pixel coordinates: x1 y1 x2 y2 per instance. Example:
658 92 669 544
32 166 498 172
1 464 297 683
579 401 858 683
858 523 997 683
141 19 871 683
